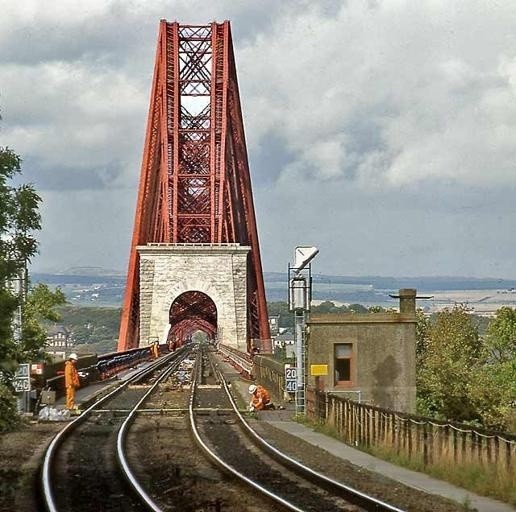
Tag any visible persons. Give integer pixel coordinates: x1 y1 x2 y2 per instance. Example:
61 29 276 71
166 338 181 352
150 341 160 359
249 385 270 410
64 353 80 410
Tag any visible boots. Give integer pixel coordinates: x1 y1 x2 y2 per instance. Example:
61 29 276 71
264 403 274 410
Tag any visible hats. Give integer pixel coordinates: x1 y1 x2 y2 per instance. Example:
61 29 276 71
248 385 256 394
68 354 77 361
